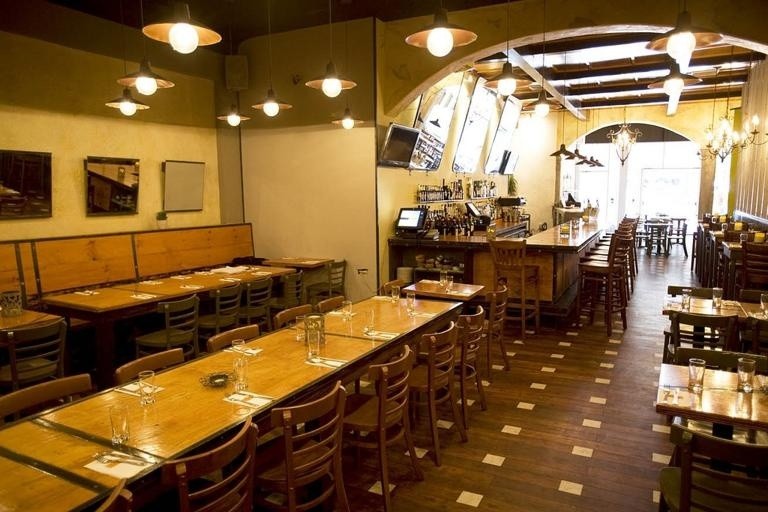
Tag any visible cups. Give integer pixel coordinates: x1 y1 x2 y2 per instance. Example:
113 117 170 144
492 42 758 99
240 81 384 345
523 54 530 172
296 271 455 363
712 289 723 309
760 293 768 312
138 370 154 405
110 407 129 443
682 289 692 309
689 357 706 390
738 359 757 392
234 358 249 390
706 213 747 245
487 223 496 239
233 340 245 362
559 207 600 237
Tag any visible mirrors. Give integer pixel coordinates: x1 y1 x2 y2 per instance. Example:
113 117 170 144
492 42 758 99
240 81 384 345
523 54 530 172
409 72 523 177
86 156 141 218
0 150 53 222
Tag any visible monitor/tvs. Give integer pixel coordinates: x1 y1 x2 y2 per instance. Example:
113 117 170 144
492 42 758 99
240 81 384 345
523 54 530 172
465 202 481 217
379 125 420 168
395 208 428 233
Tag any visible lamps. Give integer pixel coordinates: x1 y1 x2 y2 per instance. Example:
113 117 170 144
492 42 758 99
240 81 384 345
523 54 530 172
403 0 563 120
607 106 644 166
551 64 603 170
100 1 357 126
646 0 723 96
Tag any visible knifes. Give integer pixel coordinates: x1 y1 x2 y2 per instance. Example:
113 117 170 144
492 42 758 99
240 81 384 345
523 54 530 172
104 451 158 466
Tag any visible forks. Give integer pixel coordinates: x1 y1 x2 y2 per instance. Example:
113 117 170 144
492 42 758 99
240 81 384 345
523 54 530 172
95 453 156 467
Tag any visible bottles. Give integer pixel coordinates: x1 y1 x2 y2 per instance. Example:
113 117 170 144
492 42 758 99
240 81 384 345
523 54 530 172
421 206 529 233
416 179 497 200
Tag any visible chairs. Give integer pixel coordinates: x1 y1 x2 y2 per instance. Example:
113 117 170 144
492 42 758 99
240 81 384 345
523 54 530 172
488 239 540 340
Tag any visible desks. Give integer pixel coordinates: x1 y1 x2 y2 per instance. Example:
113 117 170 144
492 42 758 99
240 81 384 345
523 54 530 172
554 207 586 225
